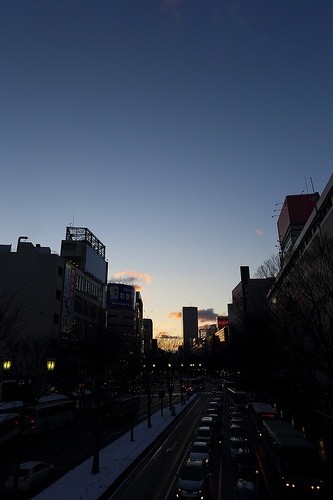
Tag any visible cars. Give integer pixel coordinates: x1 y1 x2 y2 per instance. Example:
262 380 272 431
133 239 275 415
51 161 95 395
6 459 56 496
176 363 333 499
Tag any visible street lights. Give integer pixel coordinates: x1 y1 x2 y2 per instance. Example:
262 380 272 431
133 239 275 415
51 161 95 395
143 362 156 429
164 362 174 415
2 357 59 499
179 364 188 404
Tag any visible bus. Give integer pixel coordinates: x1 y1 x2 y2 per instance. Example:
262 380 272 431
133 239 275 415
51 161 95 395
20 398 76 438
